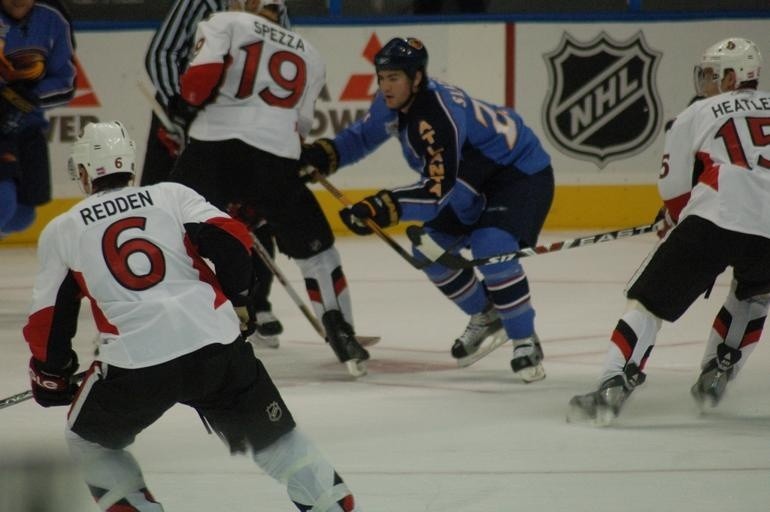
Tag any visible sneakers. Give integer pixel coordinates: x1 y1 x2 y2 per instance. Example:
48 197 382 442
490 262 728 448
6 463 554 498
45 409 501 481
451 301 503 358
510 332 544 373
322 309 369 364
567 363 647 420
691 343 742 409
254 311 282 336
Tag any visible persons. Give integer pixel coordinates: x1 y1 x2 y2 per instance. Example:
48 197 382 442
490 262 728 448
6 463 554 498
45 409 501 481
296 35 555 370
1 1 76 237
23 122 356 512
570 39 769 415
169 1 374 362
142 0 292 337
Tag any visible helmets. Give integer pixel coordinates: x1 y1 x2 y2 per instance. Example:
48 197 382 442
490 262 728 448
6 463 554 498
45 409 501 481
65 120 136 197
693 37 761 97
373 37 428 87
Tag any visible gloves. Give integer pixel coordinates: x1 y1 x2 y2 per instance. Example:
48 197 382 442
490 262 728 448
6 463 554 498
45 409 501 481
338 189 402 235
231 266 257 339
29 350 80 408
157 92 205 159
297 138 340 183
655 205 675 239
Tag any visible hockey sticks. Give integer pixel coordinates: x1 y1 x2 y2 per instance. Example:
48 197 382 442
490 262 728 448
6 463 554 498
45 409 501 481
252 231 380 346
405 224 663 268
